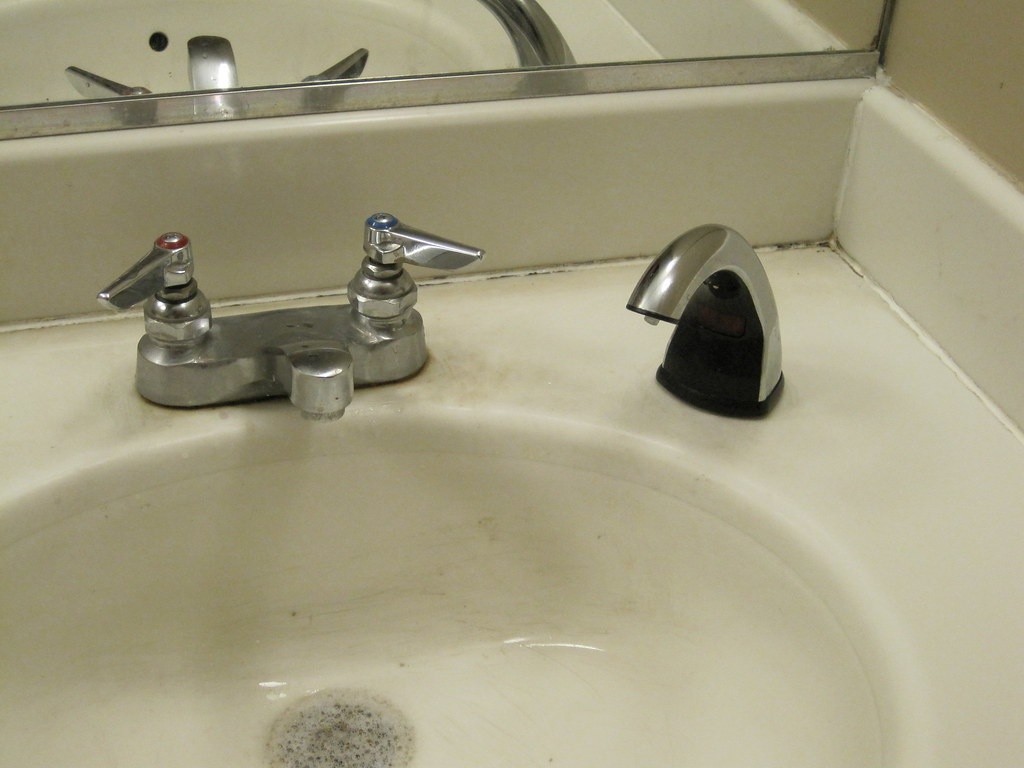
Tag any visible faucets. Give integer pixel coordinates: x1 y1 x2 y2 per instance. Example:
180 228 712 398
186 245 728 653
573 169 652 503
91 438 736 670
185 32 240 92
628 221 787 426
62 32 373 129
273 337 355 423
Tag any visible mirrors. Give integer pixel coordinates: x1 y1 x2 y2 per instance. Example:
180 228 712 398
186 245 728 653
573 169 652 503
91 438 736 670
0 0 896 140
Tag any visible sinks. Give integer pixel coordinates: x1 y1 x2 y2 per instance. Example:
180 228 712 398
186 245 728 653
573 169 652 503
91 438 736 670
2 1 521 102
0 400 919 768
